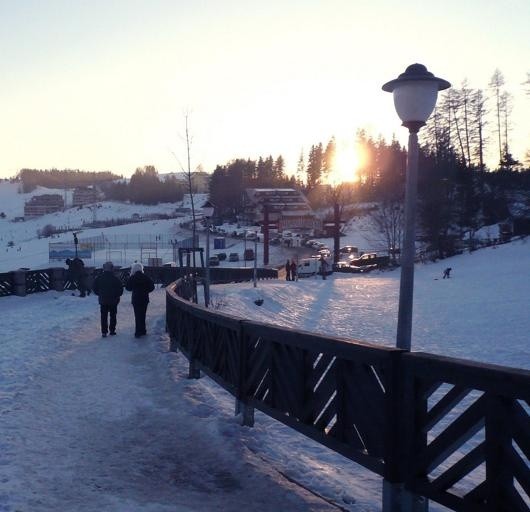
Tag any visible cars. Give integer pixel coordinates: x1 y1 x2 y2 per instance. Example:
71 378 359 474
209 249 255 266
209 222 282 244
282 231 330 257
340 246 359 253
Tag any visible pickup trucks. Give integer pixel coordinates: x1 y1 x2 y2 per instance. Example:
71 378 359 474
349 253 390 265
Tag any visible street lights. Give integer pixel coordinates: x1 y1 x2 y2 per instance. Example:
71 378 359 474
381 62 451 352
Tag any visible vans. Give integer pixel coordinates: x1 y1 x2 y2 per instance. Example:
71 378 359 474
295 257 333 277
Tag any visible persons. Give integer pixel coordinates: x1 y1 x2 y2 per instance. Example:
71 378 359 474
286 257 330 281
126 263 155 337
443 267 452 279
91 262 124 337
66 258 92 297
